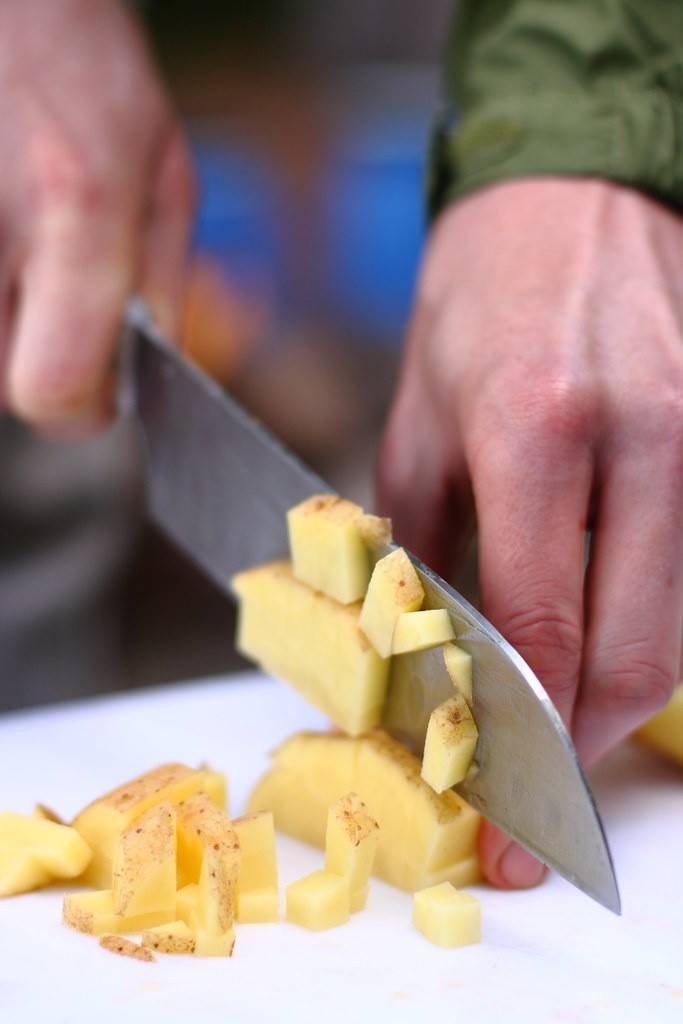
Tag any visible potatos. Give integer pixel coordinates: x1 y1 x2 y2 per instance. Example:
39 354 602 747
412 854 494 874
4 491 480 964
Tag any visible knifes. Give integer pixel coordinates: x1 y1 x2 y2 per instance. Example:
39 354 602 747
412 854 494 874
100 274 622 911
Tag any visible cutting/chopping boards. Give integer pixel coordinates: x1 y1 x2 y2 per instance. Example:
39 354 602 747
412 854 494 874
2 647 681 961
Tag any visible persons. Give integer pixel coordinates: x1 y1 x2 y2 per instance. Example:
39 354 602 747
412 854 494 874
0 0 682 893
